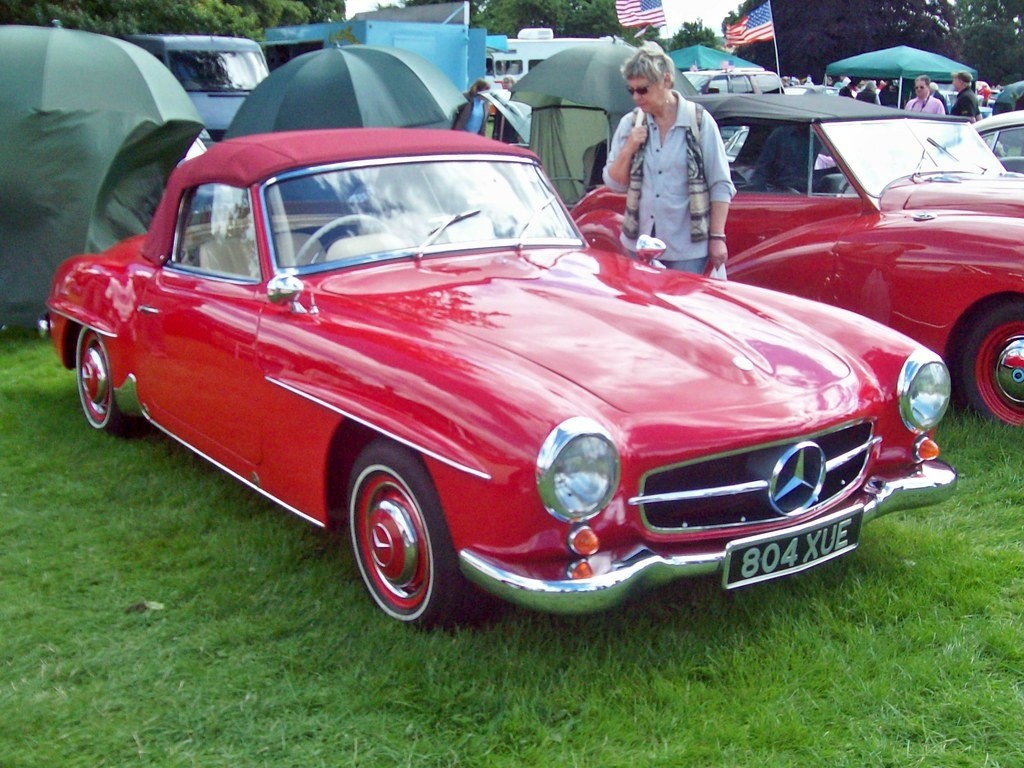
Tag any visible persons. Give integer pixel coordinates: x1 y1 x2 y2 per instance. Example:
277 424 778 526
452 80 490 134
602 49 737 274
492 75 517 143
783 72 990 123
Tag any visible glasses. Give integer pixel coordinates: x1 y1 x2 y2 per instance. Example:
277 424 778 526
915 86 924 89
627 85 649 95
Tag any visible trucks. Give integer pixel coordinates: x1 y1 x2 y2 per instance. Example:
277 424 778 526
128 33 273 143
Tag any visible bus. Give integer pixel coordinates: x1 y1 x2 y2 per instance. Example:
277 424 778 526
483 28 629 96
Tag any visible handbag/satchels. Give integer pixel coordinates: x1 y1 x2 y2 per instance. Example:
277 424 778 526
709 262 727 280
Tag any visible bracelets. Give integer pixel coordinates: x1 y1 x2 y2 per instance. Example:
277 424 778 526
710 233 726 237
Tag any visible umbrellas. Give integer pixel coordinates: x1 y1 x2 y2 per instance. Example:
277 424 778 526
223 41 470 139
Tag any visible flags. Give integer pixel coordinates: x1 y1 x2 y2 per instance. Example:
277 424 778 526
615 0 667 28
725 2 774 46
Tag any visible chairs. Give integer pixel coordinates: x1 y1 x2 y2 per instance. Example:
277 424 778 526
821 173 854 193
198 234 327 280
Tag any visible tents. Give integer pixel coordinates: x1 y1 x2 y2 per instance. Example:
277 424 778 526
0 18 204 322
508 42 699 208
826 46 978 109
667 44 762 69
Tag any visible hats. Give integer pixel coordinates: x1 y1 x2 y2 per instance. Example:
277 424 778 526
950 71 973 82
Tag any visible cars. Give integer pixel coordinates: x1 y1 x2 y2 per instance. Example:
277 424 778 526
816 84 840 95
930 84 1024 174
683 66 787 94
36 125 960 623
785 86 815 95
569 94 1024 431
